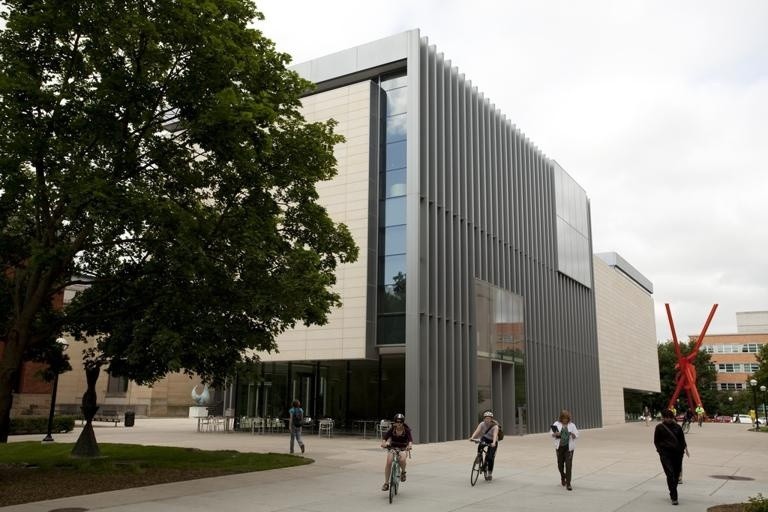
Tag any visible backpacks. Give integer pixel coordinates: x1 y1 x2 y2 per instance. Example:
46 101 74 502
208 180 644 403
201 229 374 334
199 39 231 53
293 408 303 427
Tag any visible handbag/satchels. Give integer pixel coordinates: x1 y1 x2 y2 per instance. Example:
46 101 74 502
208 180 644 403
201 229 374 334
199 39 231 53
491 420 504 440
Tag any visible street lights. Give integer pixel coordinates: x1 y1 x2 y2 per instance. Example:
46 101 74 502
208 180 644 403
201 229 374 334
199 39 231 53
727 397 733 422
750 378 761 429
42 338 69 441
759 385 767 425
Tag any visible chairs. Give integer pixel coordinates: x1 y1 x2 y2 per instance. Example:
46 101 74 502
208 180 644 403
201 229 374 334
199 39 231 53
194 415 335 438
376 422 391 439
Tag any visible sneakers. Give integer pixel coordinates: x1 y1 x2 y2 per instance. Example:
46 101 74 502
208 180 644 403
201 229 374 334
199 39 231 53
566 480 572 490
488 473 492 480
562 477 565 485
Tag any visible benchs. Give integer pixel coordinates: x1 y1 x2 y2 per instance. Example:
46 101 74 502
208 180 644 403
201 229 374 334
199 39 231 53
81 410 120 427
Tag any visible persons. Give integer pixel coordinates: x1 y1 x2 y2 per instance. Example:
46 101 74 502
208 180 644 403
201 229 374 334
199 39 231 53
289 399 305 453
654 409 685 505
749 407 756 426
470 411 498 481
550 410 578 490
381 413 413 491
642 398 705 429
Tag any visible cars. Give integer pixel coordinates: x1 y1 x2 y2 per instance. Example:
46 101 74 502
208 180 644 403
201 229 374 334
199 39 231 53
758 417 768 425
711 415 733 423
638 415 652 421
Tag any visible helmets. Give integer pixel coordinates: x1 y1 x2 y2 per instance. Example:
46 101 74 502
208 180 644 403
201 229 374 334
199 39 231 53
394 414 404 420
484 412 493 417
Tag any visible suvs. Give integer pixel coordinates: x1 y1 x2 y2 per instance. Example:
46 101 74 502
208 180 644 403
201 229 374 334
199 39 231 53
731 413 765 424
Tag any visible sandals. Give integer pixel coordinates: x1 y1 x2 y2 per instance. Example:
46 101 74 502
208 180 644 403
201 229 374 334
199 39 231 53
382 484 389 490
401 472 406 482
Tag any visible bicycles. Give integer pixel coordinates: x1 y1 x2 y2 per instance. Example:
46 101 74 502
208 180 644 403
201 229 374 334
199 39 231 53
696 411 704 428
469 439 495 486
681 417 695 433
380 444 412 503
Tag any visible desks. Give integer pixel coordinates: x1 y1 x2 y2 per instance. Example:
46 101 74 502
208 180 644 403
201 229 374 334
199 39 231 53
354 420 376 438
